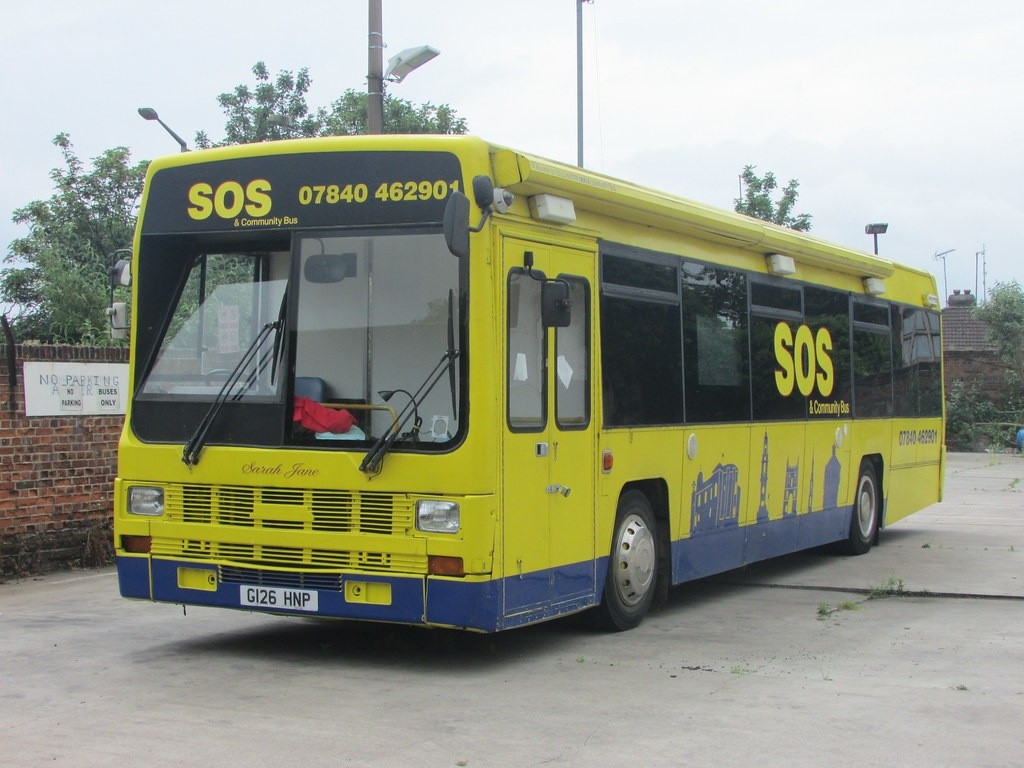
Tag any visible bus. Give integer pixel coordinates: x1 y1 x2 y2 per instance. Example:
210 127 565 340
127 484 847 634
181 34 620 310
105 135 947 635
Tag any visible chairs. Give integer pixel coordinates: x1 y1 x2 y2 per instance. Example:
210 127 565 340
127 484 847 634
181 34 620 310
292 377 328 404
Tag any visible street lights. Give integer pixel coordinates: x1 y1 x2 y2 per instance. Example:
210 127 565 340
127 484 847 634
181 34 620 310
367 1 441 135
865 222 888 257
